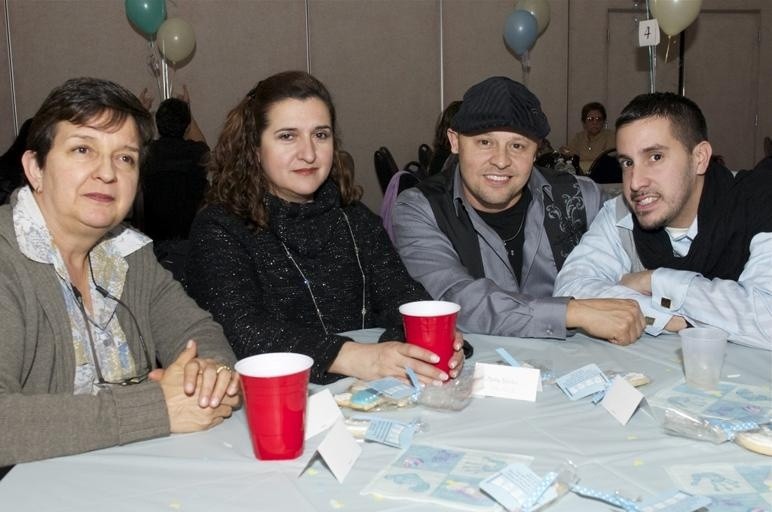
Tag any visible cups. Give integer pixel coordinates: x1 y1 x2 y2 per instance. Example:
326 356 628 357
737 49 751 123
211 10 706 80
399 299 462 381
233 352 314 462
680 329 731 390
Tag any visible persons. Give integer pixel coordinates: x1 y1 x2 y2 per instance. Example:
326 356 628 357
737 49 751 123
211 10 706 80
179 72 475 387
551 92 771 354
390 76 647 346
567 103 623 161
532 140 586 175
0 74 243 472
132 84 210 235
426 99 464 178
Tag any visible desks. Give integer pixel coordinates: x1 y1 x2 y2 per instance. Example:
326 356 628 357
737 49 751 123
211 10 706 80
1 318 769 512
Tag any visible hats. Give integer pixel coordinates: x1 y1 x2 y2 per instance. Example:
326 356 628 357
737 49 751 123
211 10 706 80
448 75 550 141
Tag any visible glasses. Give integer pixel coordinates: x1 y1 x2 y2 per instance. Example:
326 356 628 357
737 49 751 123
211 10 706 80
89 361 156 389
586 114 603 122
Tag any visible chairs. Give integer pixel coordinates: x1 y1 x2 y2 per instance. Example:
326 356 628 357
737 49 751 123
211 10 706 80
371 144 415 197
586 148 622 184
419 143 436 168
378 146 402 179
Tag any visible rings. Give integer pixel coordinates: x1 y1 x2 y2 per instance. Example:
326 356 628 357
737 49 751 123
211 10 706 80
217 365 231 374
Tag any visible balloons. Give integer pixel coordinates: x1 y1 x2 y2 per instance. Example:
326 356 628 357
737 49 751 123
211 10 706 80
503 9 539 60
648 0 702 37
125 0 166 37
156 18 195 65
515 0 551 32
